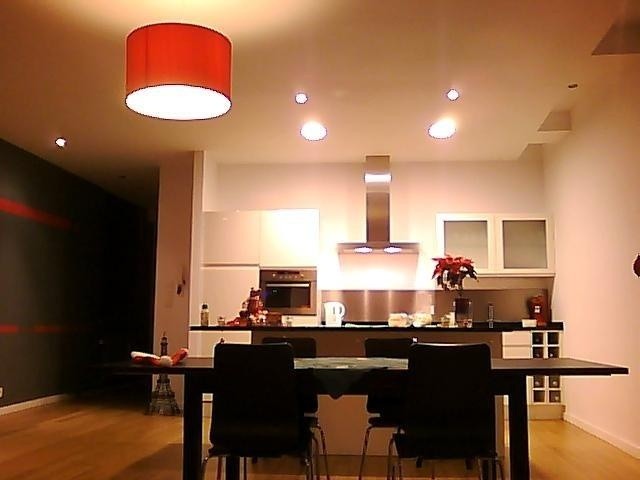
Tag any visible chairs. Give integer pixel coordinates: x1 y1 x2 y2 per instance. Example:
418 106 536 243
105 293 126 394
386 338 496 480
201 344 313 480
358 337 419 479
250 335 330 480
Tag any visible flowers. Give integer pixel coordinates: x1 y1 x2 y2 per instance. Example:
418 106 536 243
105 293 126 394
431 253 479 298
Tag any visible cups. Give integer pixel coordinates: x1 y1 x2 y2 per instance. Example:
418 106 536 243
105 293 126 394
324 302 345 327
217 315 225 324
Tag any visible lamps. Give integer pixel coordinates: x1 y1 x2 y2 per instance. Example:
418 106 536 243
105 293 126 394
124 22 233 123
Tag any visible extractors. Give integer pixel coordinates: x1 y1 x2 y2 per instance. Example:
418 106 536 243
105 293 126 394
335 155 419 274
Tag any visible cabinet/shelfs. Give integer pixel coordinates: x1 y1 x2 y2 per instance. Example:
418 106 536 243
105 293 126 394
502 329 563 406
202 207 321 322
434 211 556 278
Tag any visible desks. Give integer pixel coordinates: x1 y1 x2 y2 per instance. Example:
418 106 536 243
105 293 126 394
128 355 628 480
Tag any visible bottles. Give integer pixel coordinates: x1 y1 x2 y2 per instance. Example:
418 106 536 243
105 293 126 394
201 305 209 326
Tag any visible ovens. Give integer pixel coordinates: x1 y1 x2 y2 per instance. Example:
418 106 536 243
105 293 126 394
258 268 317 316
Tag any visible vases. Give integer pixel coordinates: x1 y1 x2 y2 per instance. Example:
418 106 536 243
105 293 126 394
454 297 474 329
